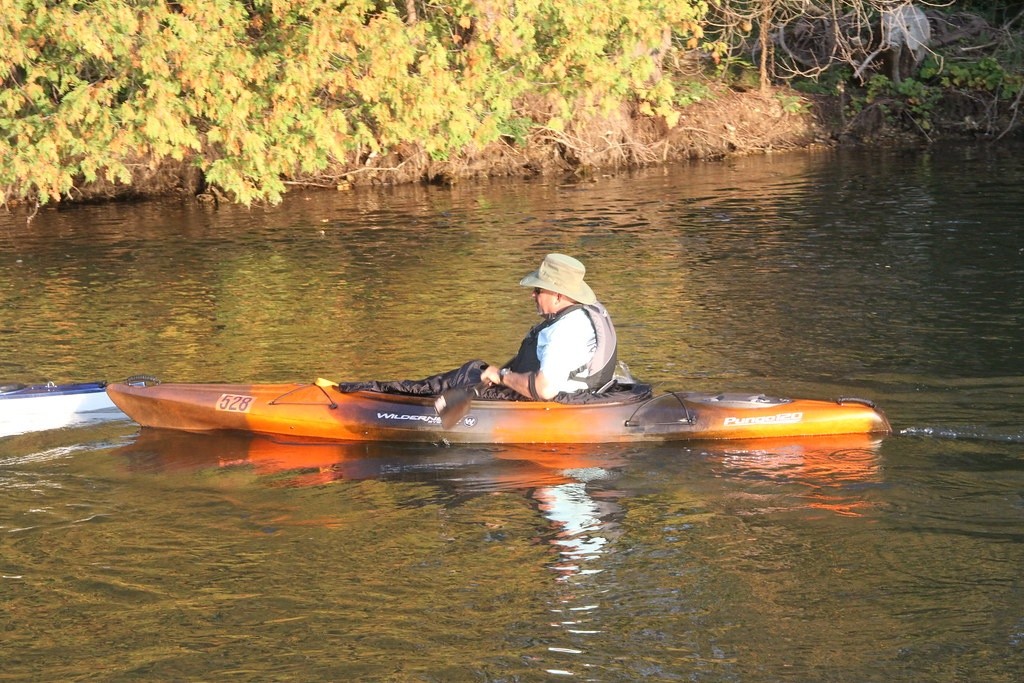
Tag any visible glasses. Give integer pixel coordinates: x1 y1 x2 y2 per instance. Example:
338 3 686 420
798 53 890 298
535 287 540 294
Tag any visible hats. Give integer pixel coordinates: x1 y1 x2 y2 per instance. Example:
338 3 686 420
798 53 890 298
520 254 596 305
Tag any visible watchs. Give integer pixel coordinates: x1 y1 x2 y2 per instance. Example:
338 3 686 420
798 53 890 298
499 368 512 386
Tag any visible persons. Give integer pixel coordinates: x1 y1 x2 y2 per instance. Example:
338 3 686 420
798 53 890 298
481 252 617 400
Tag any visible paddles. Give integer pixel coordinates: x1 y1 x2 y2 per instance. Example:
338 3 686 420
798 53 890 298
430 357 514 431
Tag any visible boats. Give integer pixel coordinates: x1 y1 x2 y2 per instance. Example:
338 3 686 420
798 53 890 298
103 375 891 453
0 381 147 438
